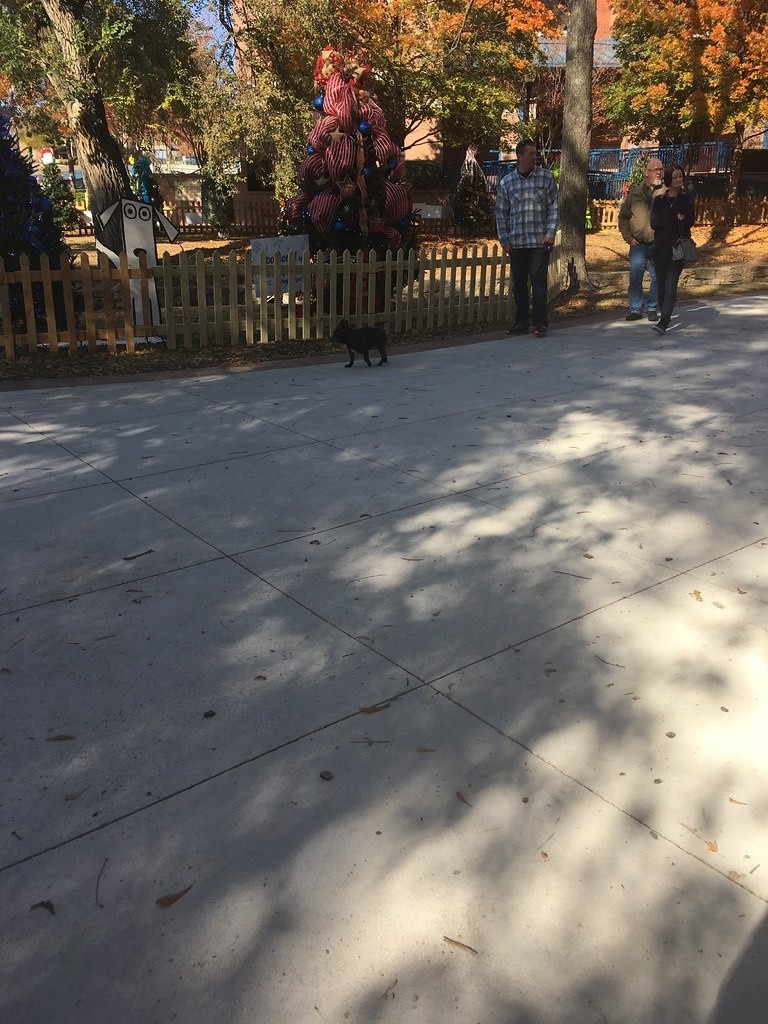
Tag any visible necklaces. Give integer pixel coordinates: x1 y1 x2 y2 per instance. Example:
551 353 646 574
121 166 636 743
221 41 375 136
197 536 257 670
668 197 676 207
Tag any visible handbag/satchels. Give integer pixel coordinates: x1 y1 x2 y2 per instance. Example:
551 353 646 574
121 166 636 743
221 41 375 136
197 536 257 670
670 217 699 265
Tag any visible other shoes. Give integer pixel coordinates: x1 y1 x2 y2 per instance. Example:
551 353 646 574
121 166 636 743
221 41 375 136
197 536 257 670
506 323 530 335
652 320 667 335
626 313 643 320
646 311 659 321
532 328 546 336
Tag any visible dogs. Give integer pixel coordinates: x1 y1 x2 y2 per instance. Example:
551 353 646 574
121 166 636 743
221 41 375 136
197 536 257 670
330 319 390 368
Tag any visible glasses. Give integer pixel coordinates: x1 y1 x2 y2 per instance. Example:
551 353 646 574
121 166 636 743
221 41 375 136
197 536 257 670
648 168 663 173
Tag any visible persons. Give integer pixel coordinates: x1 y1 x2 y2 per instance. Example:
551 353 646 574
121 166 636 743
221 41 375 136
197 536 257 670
650 165 695 335
496 140 560 337
618 159 670 321
